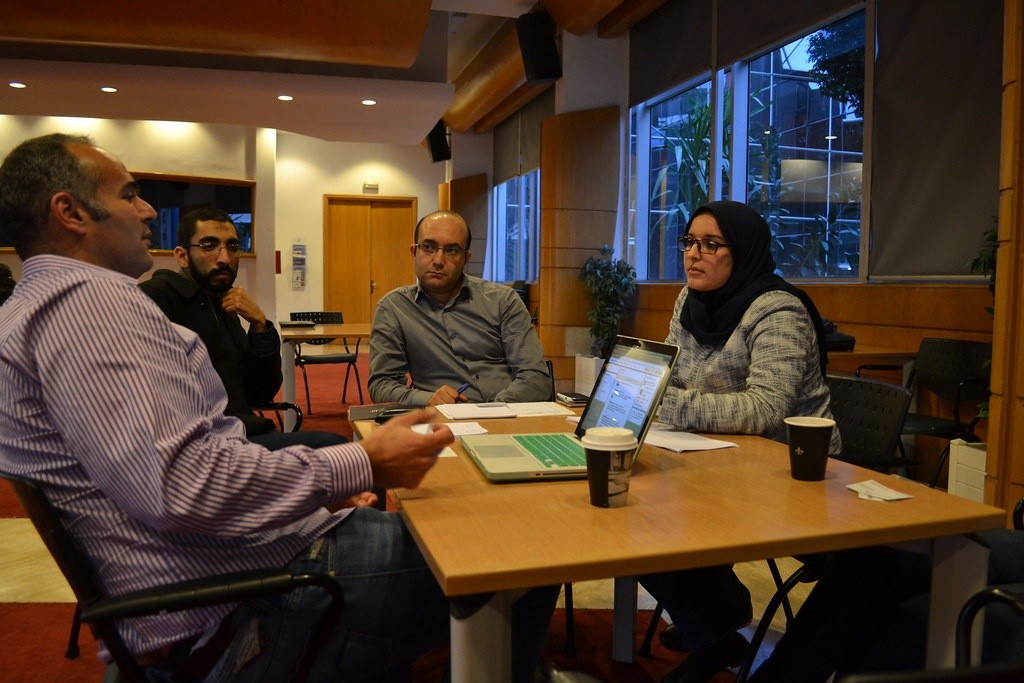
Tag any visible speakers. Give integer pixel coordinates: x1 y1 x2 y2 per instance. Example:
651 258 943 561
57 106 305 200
515 12 563 83
428 120 452 163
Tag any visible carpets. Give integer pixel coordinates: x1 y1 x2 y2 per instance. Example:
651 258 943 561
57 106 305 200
1 353 398 518
0 602 741 682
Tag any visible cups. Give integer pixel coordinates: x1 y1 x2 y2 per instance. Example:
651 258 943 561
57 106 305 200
580 427 640 509
784 417 837 482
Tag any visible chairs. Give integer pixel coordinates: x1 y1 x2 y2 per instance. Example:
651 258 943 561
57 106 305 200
9 280 1024 683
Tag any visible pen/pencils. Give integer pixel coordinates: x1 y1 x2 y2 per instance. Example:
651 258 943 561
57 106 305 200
456 383 470 393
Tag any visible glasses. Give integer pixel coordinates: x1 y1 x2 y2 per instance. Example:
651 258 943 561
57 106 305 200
677 236 729 254
414 244 471 257
180 242 246 257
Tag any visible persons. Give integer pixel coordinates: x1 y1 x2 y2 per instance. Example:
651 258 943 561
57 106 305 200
1 134 563 683
138 210 349 450
367 210 552 405
640 204 842 665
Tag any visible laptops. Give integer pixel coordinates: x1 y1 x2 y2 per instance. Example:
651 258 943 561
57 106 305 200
461 334 682 483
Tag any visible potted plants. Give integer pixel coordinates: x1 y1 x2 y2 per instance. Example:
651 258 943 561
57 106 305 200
574 244 637 398
948 216 998 504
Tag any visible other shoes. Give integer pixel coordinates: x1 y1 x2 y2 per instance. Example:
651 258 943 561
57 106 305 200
665 632 751 683
659 601 754 652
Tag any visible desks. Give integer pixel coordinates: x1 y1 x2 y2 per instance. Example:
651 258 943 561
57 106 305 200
828 346 919 480
280 324 373 337
347 404 1007 683
